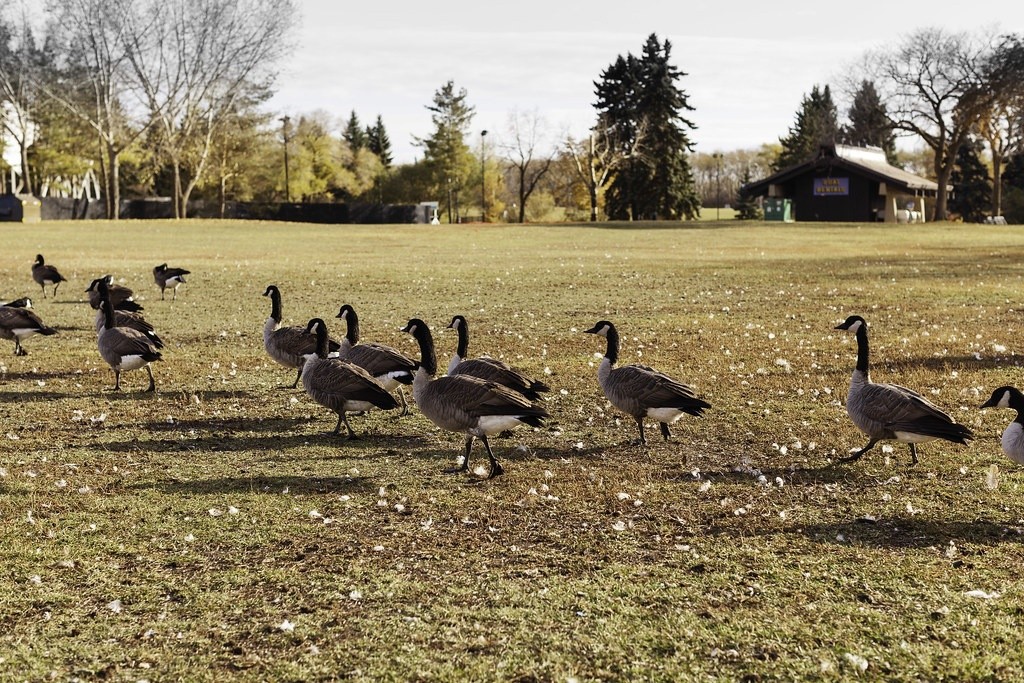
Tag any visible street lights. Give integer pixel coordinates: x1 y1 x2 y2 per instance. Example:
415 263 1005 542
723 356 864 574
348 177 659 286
712 153 725 220
479 130 488 224
277 115 291 202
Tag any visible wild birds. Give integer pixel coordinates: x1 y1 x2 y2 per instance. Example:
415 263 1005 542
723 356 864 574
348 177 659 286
334 304 425 421
583 320 714 448
399 316 550 481
299 316 399 440
829 315 975 473
977 386 1024 465
32 253 68 298
153 263 191 302
84 275 165 395
262 284 342 389
443 314 554 402
0 296 60 357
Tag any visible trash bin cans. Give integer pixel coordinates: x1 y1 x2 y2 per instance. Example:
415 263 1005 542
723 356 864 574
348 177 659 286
763 198 793 222
